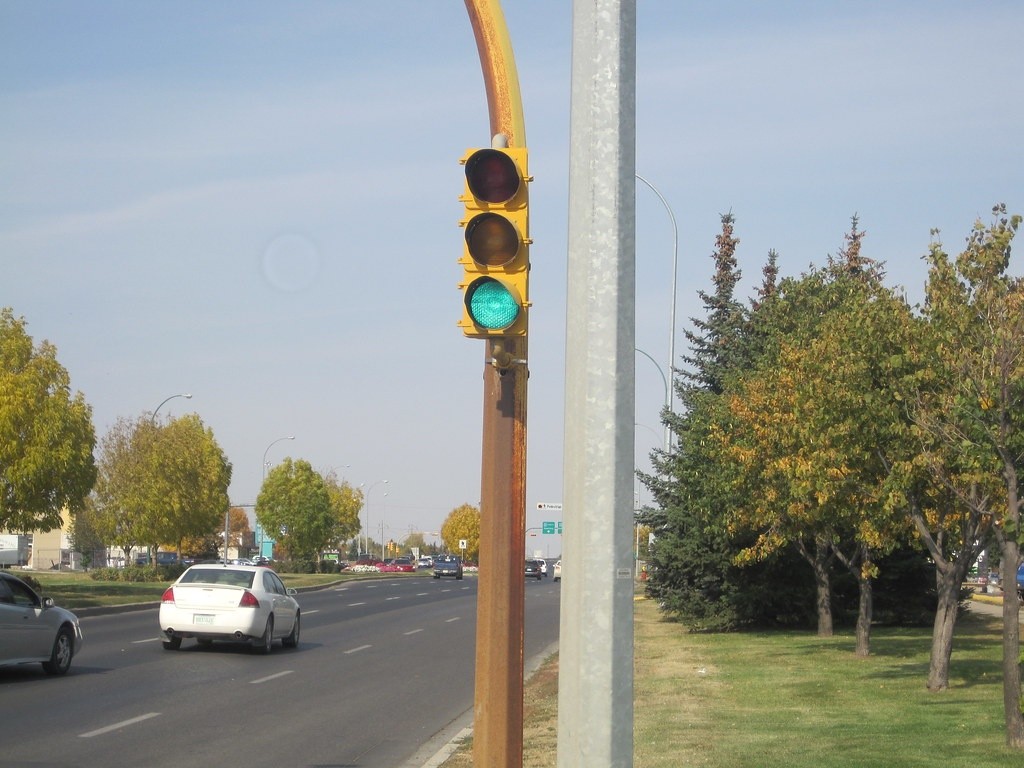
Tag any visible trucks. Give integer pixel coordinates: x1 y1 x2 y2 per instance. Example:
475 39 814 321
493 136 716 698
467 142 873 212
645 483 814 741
0 532 29 567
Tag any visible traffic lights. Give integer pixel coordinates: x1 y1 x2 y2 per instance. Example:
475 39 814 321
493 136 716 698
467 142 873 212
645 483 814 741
455 149 534 336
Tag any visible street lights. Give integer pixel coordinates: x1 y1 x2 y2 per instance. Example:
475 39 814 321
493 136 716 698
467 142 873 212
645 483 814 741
149 392 196 430
262 435 293 491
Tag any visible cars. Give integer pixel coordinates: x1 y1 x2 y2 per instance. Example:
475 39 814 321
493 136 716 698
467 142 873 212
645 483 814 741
132 549 280 568
318 552 441 579
552 559 561 581
536 559 547 576
434 554 463 580
155 564 303 654
524 559 541 579
0 572 83 677
